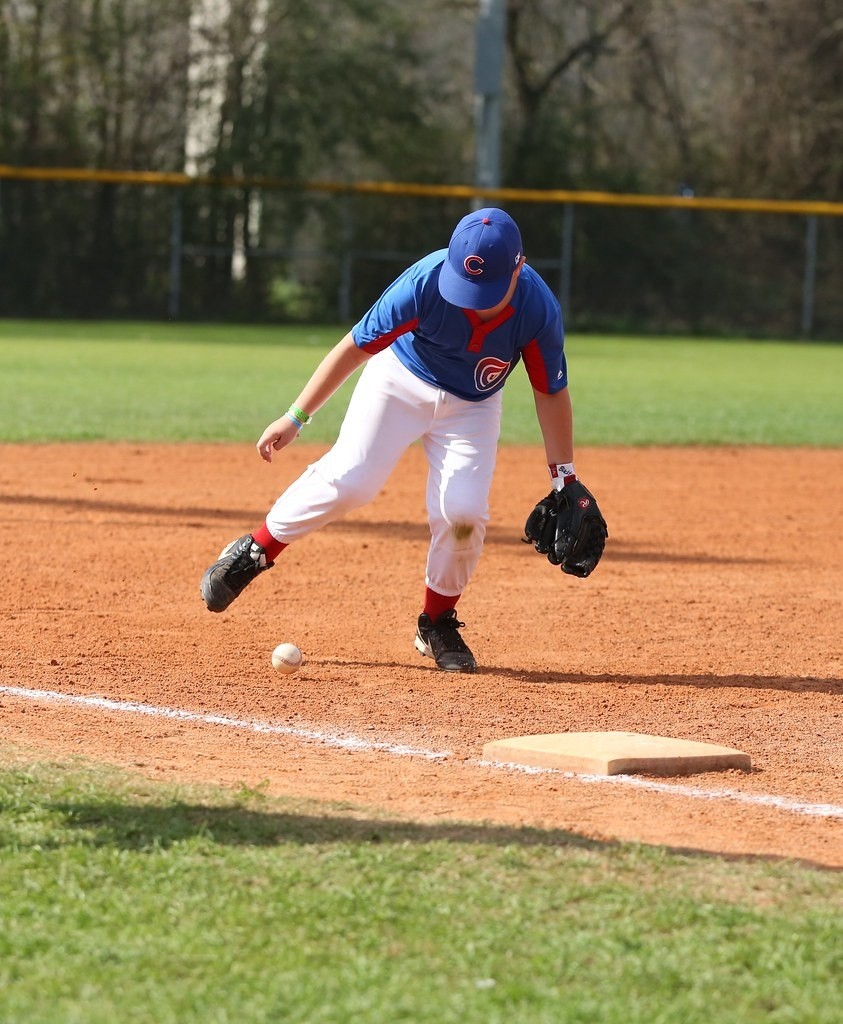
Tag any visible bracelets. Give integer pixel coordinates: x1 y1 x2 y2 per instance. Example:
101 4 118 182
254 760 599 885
547 462 578 492
288 403 312 424
285 412 303 430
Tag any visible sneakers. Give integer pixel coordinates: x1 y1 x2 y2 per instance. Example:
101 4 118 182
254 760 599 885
414 608 476 673
199 532 277 613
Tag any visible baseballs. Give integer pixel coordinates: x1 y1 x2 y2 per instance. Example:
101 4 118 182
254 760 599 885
271 643 302 674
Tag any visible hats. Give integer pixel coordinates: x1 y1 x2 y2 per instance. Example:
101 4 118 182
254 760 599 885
437 208 523 310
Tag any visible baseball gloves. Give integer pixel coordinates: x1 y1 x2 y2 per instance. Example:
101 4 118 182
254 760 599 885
520 480 609 578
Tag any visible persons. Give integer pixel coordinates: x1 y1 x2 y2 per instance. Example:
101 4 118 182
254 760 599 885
202 207 609 673
